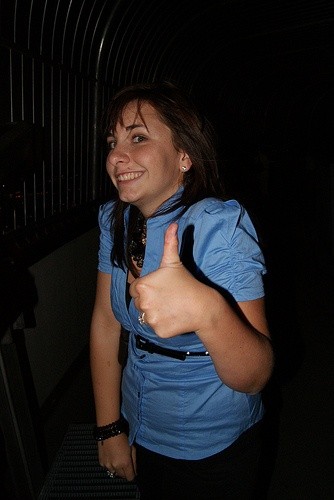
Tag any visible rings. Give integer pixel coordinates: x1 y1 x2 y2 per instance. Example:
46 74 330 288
138 313 147 325
107 470 118 479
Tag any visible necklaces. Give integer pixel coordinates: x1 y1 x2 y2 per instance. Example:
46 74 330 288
128 216 146 268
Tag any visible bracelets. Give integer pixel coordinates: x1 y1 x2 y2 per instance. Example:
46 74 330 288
92 419 125 441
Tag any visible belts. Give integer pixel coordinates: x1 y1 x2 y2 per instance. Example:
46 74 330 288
134 334 210 360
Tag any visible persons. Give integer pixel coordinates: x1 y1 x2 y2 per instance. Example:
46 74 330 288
88 80 276 500
